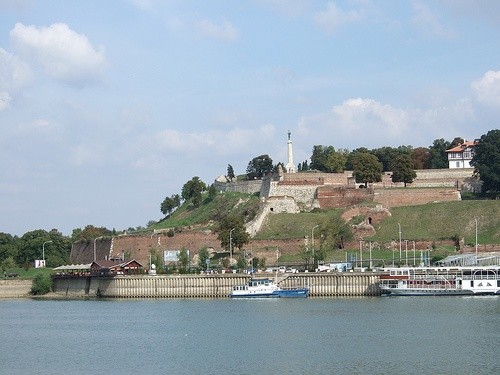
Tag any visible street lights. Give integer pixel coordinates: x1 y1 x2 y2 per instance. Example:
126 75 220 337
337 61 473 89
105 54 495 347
230 228 236 266
474 218 477 266
398 223 402 261
312 225 319 271
43 240 53 260
94 236 104 261
275 249 279 273
404 240 409 267
412 240 416 266
420 249 432 268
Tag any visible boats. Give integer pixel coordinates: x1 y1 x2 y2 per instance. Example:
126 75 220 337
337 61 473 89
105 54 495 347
378 267 500 297
230 277 309 298
